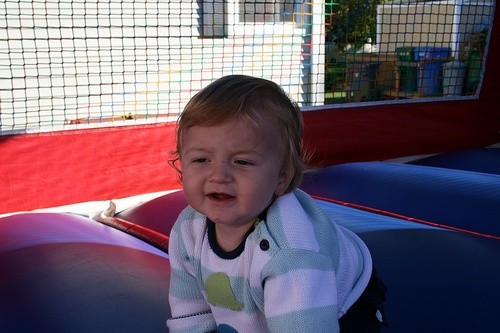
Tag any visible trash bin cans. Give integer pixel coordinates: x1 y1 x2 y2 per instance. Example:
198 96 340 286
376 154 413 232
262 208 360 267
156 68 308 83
396 46 453 97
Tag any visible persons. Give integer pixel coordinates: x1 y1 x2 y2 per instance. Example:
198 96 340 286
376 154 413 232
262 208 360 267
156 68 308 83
165 74 389 333
